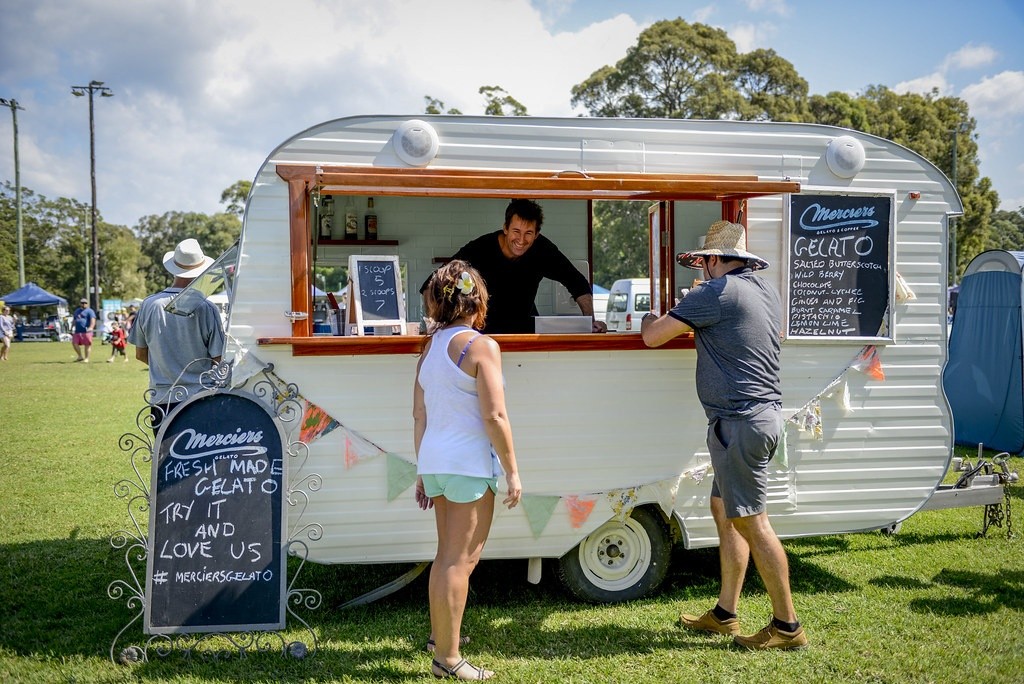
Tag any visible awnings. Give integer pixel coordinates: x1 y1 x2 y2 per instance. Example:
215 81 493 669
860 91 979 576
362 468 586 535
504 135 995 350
309 170 802 310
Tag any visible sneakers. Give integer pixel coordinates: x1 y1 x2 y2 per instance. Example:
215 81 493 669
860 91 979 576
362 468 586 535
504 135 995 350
680 609 742 636
734 621 809 651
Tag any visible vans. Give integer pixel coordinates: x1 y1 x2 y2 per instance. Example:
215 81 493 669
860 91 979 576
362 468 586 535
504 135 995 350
205 296 228 332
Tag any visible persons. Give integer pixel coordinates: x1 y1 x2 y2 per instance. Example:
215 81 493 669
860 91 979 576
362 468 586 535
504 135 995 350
106 321 128 363
128 237 224 439
116 305 137 329
418 200 609 333
71 299 96 362
642 221 808 651
413 260 520 680
0 306 17 361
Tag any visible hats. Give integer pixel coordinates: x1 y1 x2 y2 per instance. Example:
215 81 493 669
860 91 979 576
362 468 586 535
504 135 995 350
80 298 88 303
163 239 215 279
675 221 769 270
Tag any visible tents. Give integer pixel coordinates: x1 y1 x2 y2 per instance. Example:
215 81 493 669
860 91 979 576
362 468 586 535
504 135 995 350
335 287 347 296
0 283 69 319
943 250 1024 459
312 285 328 296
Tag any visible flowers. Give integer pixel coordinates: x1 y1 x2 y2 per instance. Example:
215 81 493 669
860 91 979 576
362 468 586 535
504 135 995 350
455 272 475 295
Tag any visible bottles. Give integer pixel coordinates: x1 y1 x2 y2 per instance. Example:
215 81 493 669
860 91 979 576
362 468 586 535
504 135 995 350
365 196 378 240
320 195 334 241
344 196 357 239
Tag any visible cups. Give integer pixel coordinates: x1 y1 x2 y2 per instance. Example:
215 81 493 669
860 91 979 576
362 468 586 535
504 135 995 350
328 308 346 336
374 326 392 335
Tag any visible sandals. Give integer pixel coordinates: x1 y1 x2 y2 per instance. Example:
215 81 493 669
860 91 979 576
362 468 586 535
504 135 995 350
432 659 493 681
427 636 467 649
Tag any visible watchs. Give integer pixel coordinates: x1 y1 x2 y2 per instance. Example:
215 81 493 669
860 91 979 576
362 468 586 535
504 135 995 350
642 313 657 320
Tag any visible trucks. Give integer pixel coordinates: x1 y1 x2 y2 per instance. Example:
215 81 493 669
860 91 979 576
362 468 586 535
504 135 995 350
226 110 967 603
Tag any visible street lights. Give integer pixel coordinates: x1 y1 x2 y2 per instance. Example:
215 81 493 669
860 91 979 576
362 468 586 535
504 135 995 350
0 95 29 326
69 80 114 318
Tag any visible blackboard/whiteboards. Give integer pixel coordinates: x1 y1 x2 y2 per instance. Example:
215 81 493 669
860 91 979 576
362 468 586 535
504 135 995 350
786 190 894 338
146 393 283 630
355 257 402 325
